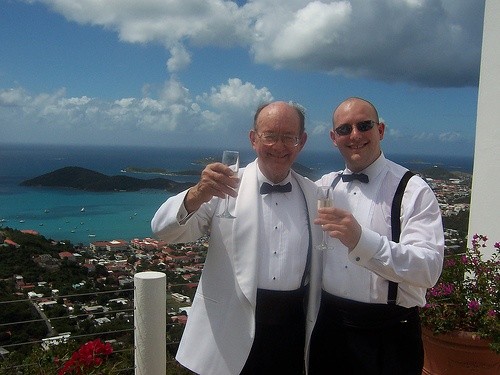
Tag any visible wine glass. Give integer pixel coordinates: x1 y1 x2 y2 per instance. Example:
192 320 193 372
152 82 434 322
315 186 333 249
216 150 239 218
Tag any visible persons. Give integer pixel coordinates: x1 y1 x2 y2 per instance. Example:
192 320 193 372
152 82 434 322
151 101 322 374
308 98 444 375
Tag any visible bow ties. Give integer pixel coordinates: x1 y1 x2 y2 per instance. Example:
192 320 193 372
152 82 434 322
338 173 369 183
260 182 292 194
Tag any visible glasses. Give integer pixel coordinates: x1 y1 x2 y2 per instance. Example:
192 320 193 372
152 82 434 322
334 120 380 136
255 132 301 146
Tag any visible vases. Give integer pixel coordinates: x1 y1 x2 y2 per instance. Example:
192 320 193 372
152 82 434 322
421 324 500 375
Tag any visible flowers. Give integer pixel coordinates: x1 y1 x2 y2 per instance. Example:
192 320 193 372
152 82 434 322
59 339 113 375
416 234 500 354
